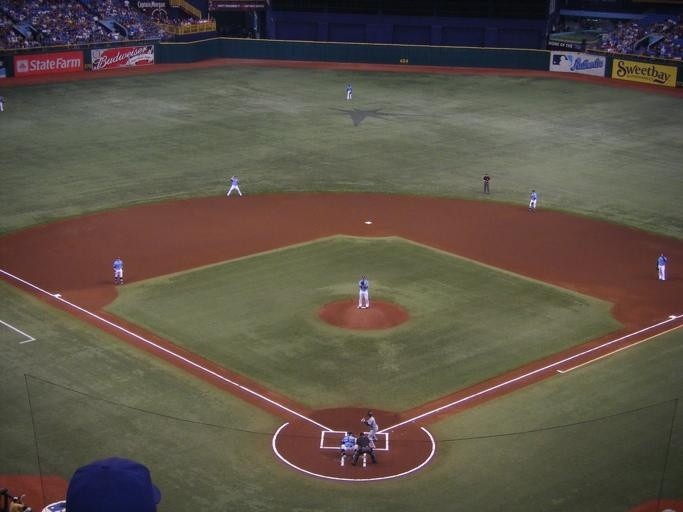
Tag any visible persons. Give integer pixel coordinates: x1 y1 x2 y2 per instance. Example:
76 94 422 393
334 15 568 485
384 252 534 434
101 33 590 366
9 493 32 512
112 255 124 285
340 411 379 466
0 0 216 49
482 173 490 194
347 84 352 100
552 17 683 60
226 175 242 198
529 189 537 209
359 275 369 309
656 252 667 281
0 95 6 112
